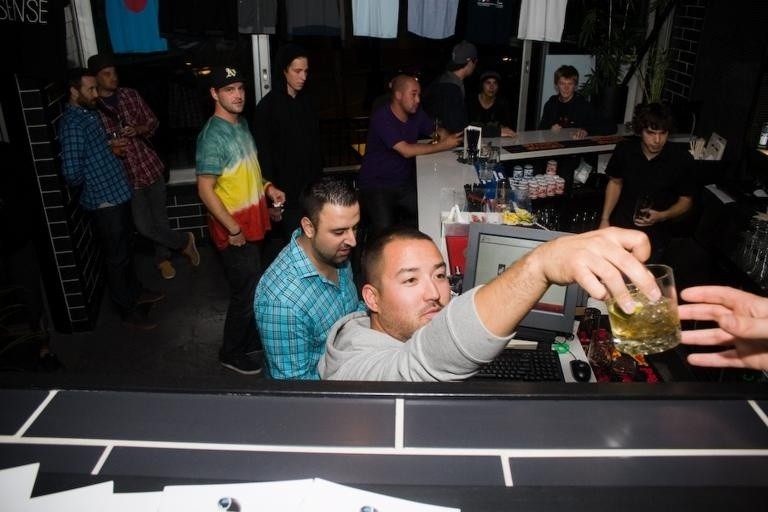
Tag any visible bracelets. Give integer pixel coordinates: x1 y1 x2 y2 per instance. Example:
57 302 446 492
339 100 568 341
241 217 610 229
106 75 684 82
263 181 273 195
230 231 244 237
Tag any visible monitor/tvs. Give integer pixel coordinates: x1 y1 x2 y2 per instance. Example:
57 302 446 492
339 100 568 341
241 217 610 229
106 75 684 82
462 220 579 350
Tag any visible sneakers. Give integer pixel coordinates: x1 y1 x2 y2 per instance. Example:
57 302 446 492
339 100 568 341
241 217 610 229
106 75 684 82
160 258 178 281
135 288 166 305
222 351 263 376
183 231 200 268
122 311 156 331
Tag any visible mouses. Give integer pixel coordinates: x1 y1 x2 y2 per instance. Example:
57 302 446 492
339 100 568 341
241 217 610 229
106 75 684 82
569 358 592 382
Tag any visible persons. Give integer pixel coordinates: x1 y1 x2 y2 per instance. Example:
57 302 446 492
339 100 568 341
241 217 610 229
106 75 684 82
371 74 395 108
678 283 768 372
92 52 202 279
421 41 516 138
58 68 169 333
249 42 322 251
596 99 703 268
537 65 593 140
192 61 289 376
358 74 464 239
316 224 661 383
252 175 366 380
466 69 514 125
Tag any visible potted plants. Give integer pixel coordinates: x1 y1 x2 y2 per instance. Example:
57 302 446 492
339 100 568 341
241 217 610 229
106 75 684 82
574 11 629 137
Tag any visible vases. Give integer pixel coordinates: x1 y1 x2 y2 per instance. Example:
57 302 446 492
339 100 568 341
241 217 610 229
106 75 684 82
616 83 630 126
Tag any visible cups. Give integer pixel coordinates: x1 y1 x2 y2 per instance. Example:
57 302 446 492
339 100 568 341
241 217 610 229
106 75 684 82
632 195 650 224
577 307 611 376
604 264 682 355
508 160 565 199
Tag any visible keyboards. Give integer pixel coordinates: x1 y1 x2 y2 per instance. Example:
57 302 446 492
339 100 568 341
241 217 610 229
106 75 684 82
477 346 566 380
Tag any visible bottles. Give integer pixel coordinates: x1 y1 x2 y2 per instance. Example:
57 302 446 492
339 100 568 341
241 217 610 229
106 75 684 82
736 216 768 287
569 209 600 225
111 132 120 156
534 207 560 230
430 116 441 147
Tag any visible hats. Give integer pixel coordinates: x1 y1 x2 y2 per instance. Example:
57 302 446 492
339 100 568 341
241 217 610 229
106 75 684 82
452 41 476 63
88 55 120 71
480 66 500 79
211 58 244 87
